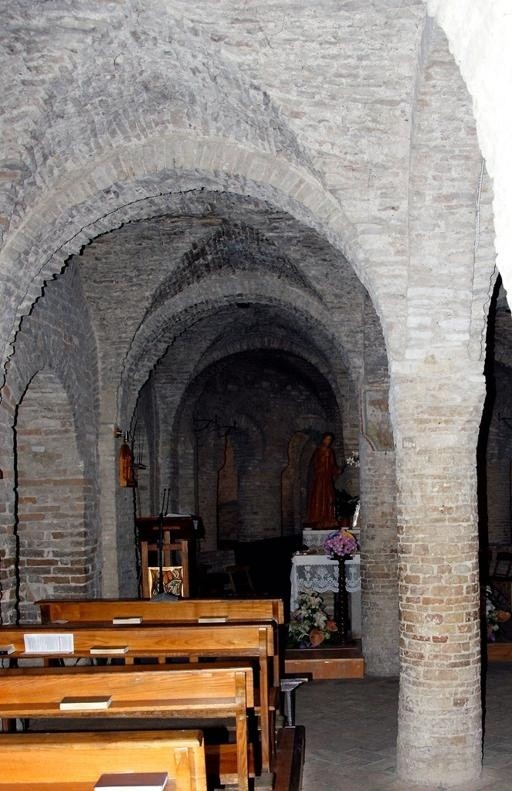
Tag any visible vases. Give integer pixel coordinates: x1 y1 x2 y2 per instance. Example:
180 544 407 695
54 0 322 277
345 477 360 497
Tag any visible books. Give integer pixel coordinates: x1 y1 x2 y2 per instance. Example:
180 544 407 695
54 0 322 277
198 616 228 623
94 771 169 790
60 696 112 710
89 645 129 654
112 617 143 624
1 645 16 656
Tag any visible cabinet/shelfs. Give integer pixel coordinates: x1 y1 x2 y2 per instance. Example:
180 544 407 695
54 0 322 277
290 554 362 641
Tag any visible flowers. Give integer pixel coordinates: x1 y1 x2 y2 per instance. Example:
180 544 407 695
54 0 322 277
319 528 359 557
287 584 338 652
343 448 361 476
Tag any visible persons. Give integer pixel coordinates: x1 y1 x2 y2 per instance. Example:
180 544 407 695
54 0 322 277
305 431 342 528
